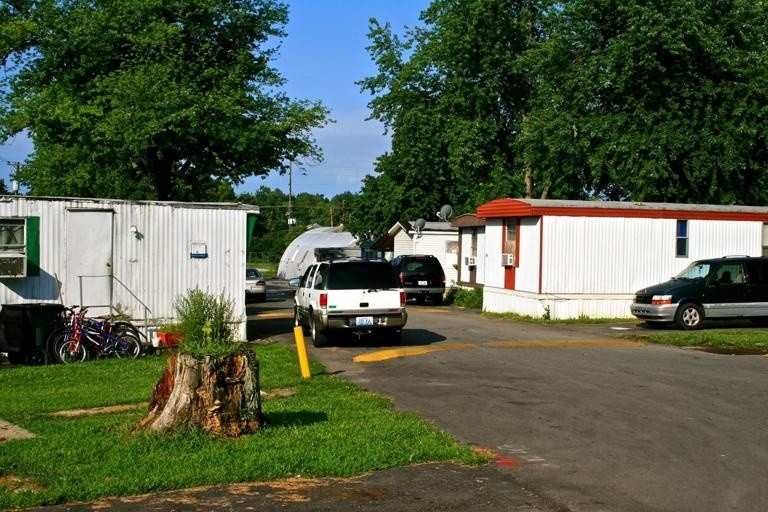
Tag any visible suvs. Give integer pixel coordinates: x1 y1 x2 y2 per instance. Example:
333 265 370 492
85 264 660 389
389 255 449 305
289 255 410 350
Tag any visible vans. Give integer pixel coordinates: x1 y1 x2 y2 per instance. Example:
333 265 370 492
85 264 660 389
627 255 768 328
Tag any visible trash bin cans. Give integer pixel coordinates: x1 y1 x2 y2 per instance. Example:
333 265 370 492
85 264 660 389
1 303 65 365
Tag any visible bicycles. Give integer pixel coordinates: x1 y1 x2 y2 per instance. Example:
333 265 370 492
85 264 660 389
46 303 141 367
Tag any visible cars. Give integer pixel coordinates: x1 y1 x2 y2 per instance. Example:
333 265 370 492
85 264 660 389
247 268 269 305
